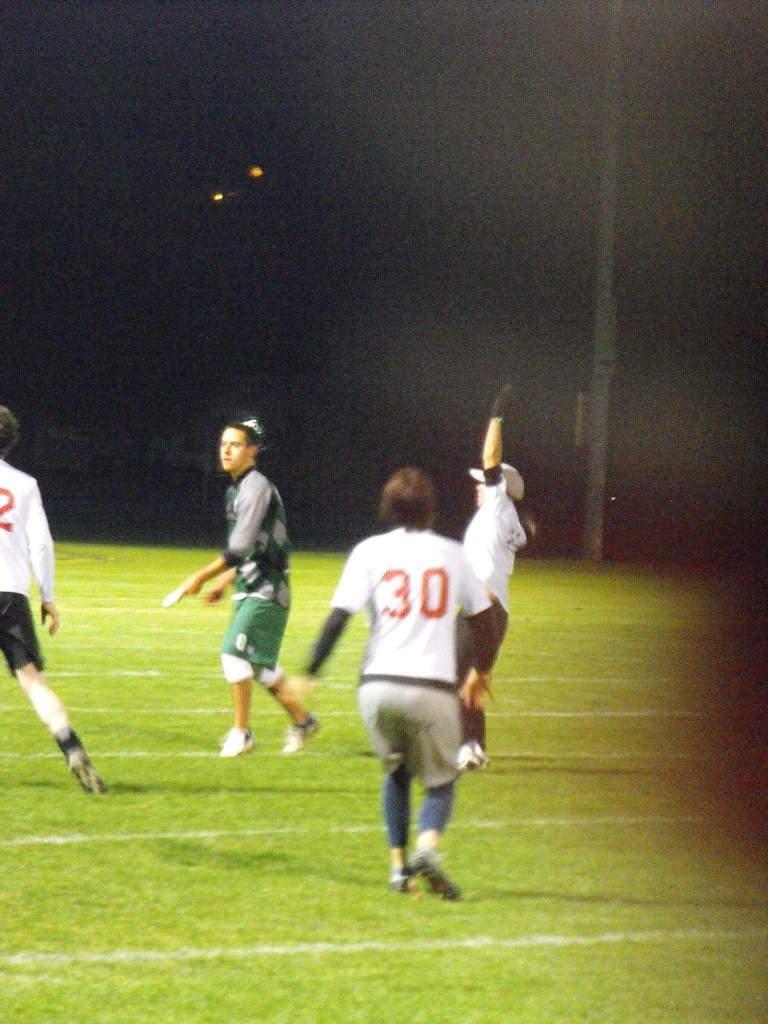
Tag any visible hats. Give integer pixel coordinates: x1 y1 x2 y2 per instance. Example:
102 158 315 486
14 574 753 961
469 463 525 501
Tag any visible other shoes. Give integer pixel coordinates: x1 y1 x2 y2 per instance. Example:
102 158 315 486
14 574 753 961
408 850 461 899
457 740 488 772
389 869 406 893
281 714 322 757
220 728 252 757
67 746 108 796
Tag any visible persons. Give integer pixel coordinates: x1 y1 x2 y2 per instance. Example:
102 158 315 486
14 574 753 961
0 405 109 795
277 467 499 905
455 384 525 774
179 419 319 758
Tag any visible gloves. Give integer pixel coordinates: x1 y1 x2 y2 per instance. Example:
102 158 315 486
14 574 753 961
490 384 514 419
